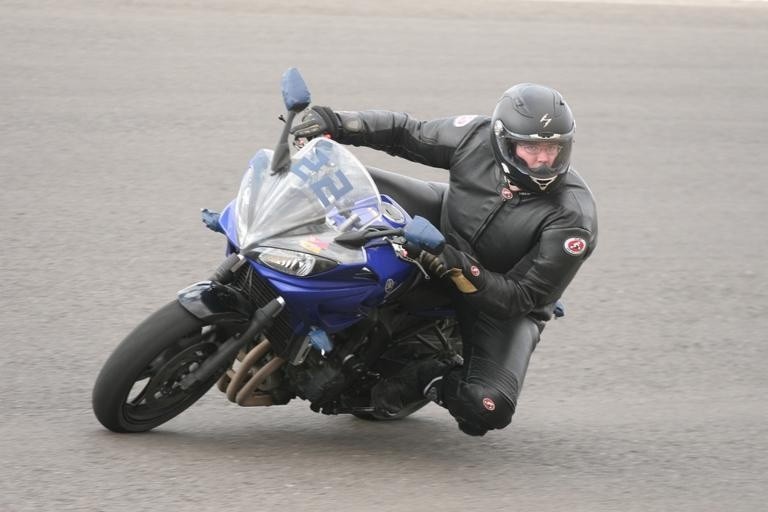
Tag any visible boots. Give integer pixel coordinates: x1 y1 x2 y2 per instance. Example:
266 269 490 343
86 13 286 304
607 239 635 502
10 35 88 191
371 359 460 413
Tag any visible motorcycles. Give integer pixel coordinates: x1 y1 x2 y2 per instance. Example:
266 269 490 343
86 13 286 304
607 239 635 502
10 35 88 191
91 67 479 434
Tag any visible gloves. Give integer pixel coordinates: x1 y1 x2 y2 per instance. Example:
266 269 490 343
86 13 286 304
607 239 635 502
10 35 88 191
419 244 485 293
290 106 363 144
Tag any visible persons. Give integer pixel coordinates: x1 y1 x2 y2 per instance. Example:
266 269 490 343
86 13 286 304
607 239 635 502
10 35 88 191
288 82 597 435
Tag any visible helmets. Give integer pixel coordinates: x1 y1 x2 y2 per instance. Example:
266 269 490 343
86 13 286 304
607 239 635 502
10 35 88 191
491 84 574 195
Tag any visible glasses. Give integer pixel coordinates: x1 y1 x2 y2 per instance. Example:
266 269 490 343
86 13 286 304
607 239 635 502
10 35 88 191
515 143 565 154
